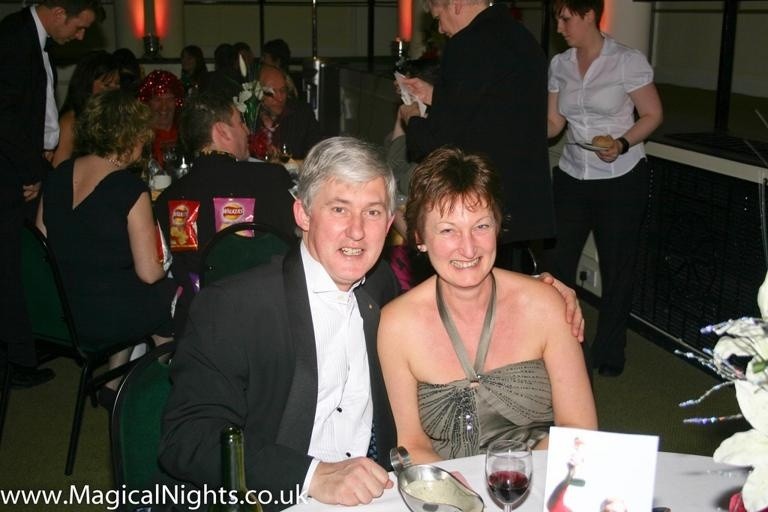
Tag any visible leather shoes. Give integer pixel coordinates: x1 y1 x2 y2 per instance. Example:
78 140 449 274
598 353 625 377
9 367 55 388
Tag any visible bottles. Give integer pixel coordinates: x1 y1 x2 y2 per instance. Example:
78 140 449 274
144 144 190 191
210 427 263 512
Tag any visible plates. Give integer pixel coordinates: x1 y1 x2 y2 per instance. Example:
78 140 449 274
580 142 609 152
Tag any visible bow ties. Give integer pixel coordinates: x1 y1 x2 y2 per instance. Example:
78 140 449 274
44 36 63 66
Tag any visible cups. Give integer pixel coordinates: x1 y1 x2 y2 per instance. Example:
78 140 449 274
388 444 486 512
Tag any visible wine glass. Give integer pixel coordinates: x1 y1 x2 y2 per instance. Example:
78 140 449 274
260 142 293 166
486 440 532 510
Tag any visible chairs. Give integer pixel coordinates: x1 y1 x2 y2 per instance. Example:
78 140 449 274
108 222 296 512
20 225 157 475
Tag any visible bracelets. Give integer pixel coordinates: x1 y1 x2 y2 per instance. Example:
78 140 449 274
618 137 629 154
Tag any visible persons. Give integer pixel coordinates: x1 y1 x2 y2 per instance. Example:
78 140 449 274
0 0 106 388
34 40 320 407
156 137 588 512
548 1 663 376
378 143 600 465
395 1 554 273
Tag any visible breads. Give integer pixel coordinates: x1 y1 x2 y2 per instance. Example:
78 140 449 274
591 134 615 148
